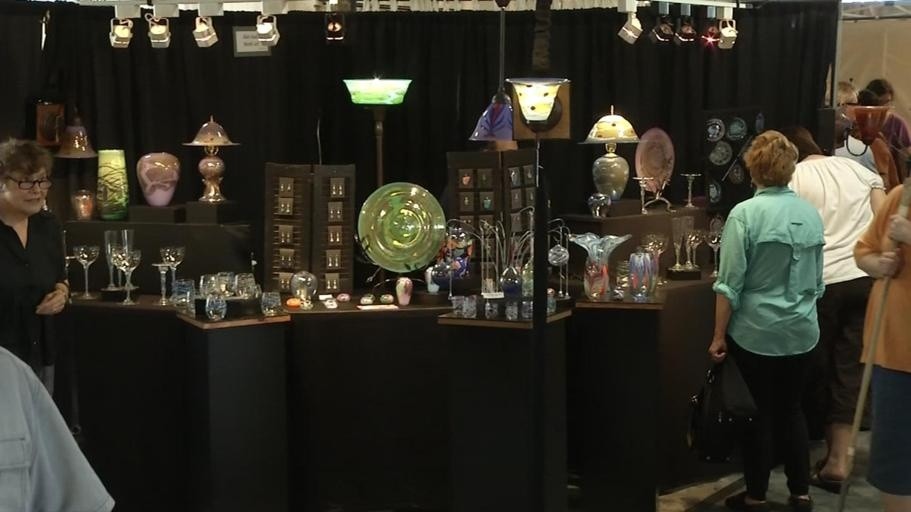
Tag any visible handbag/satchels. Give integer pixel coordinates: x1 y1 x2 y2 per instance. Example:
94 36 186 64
688 356 754 465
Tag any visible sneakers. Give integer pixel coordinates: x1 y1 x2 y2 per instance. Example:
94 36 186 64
725 491 769 512
788 494 813 512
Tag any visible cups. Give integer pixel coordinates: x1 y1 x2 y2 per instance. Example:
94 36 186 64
452 295 534 320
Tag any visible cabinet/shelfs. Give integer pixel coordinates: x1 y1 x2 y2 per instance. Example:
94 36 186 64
178 310 291 512
573 271 716 509
49 293 196 512
289 294 453 512
437 309 573 511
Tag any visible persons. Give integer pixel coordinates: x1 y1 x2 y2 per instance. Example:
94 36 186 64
1 138 72 399
707 129 826 512
1 345 117 511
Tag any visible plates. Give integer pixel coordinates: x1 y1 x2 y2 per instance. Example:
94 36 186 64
708 116 766 203
635 127 675 192
358 181 446 273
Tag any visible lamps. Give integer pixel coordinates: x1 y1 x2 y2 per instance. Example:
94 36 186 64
836 99 898 158
578 99 642 202
55 104 99 161
343 74 414 188
462 1 521 145
105 3 348 52
617 0 737 51
505 71 573 192
183 115 243 206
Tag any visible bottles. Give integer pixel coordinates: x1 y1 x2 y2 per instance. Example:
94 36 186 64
97 150 128 221
547 288 559 313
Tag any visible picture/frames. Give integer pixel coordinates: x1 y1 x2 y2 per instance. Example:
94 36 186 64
23 93 70 154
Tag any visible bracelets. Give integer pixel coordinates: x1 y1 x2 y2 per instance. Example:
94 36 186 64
55 281 71 299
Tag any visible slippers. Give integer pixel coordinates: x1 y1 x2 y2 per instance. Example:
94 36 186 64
809 458 845 494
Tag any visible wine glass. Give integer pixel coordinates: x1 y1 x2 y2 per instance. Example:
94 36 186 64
571 214 728 302
74 230 281 320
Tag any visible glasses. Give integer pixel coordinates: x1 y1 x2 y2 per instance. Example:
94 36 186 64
8 175 52 191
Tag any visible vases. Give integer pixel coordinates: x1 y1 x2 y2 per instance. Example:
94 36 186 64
95 147 133 223
137 152 184 206
